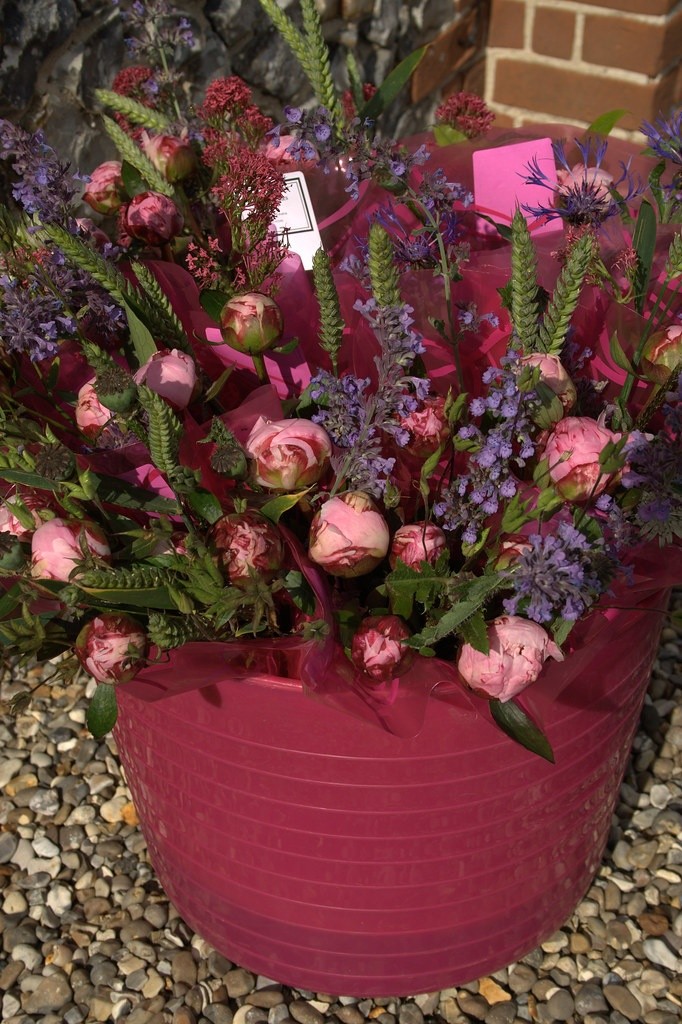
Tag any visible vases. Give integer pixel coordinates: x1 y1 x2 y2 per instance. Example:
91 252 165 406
113 546 682 996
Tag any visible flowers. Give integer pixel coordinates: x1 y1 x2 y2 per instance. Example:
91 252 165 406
0 0 682 701
